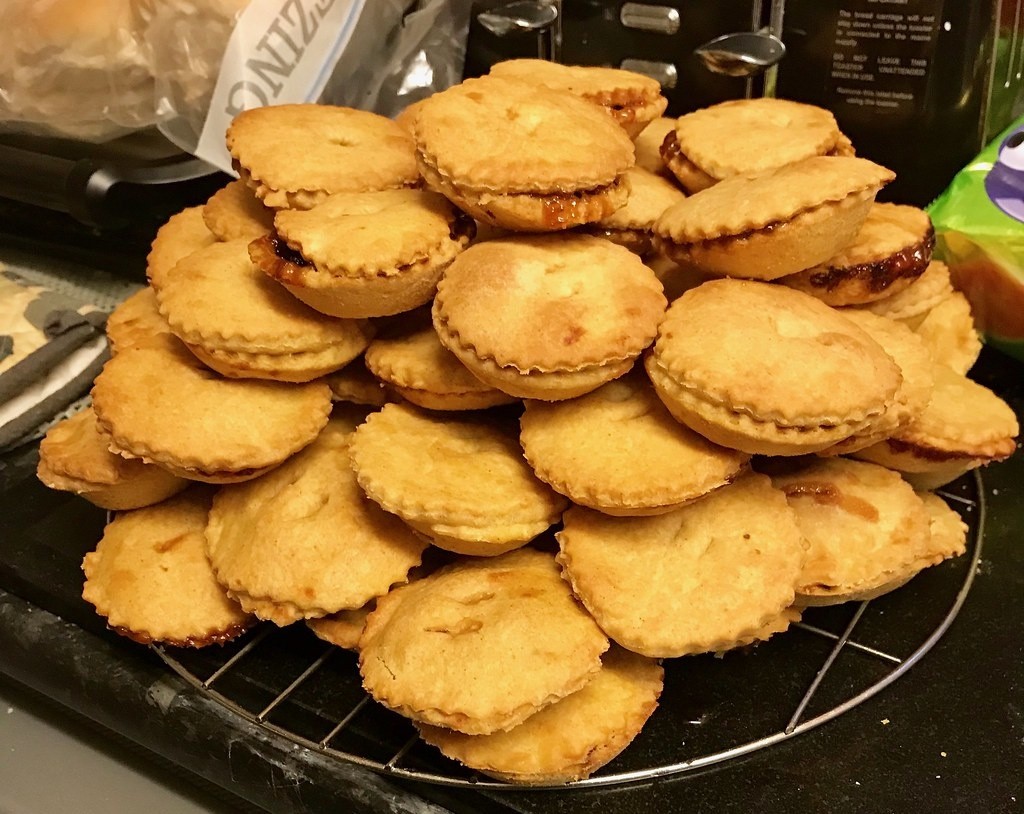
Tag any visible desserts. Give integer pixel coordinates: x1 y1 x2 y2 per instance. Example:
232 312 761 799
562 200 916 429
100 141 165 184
38 57 1019 789
